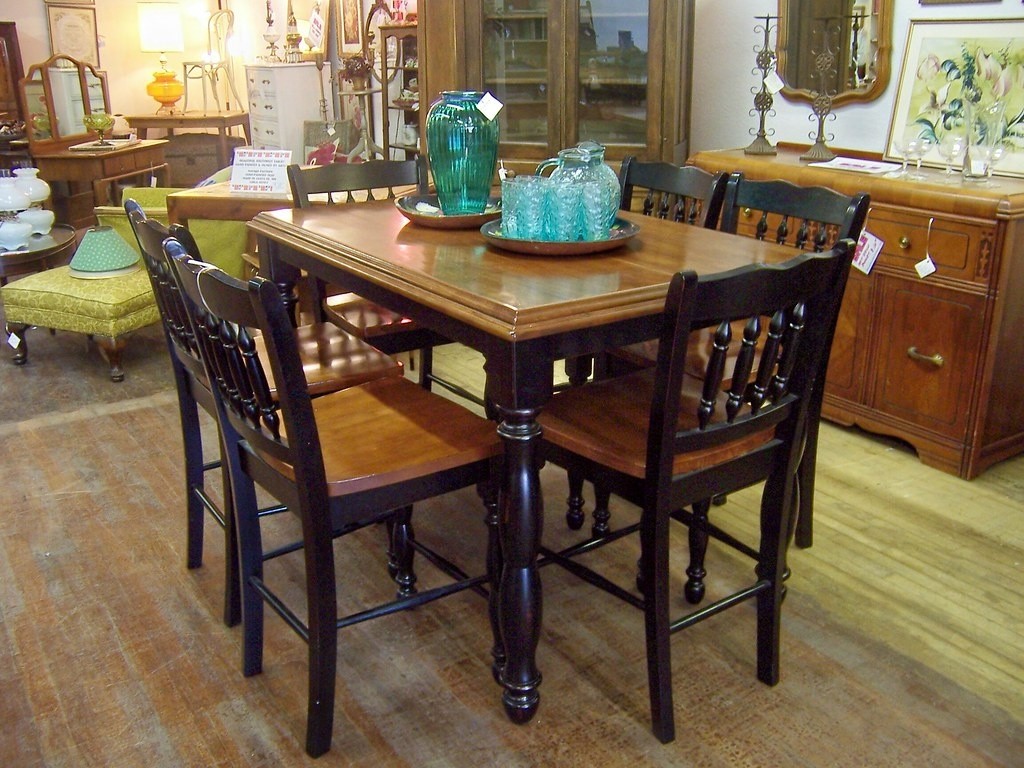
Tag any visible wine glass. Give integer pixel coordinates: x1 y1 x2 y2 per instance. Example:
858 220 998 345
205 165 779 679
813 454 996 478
893 135 962 179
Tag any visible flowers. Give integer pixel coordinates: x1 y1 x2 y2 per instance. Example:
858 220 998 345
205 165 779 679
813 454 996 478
340 55 372 81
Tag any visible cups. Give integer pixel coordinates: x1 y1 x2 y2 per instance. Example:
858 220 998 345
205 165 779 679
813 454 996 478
500 175 580 242
402 121 419 146
400 89 419 101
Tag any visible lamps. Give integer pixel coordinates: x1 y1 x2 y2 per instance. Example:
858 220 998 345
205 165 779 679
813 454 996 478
261 17 282 62
136 2 188 117
286 11 305 63
83 112 116 145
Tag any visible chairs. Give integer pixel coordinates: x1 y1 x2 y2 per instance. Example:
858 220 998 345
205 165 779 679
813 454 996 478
588 164 880 605
182 8 242 116
122 196 435 630
525 235 861 748
270 151 490 412
617 156 729 227
153 237 508 762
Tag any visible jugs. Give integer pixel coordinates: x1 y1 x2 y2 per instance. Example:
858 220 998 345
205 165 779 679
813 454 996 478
536 139 621 242
963 81 1009 182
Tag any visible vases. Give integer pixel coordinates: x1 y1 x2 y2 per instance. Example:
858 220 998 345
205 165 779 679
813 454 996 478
425 88 499 215
351 74 366 90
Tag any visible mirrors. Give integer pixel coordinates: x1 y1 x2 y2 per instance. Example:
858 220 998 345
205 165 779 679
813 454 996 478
773 0 895 108
17 53 112 146
364 0 402 84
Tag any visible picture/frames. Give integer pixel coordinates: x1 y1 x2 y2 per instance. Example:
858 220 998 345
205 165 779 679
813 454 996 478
882 14 1024 180
44 0 96 7
45 4 103 69
334 0 364 61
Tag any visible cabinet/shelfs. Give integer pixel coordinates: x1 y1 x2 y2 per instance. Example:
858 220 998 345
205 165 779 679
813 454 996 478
35 131 177 226
416 0 691 161
684 144 1024 479
381 24 422 159
242 61 333 165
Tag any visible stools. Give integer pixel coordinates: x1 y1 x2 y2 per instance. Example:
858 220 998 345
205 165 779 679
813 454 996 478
1 260 161 383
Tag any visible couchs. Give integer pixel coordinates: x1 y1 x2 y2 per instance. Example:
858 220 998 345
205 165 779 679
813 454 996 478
97 165 249 279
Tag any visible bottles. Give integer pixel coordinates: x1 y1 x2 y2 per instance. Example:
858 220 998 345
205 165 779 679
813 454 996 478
425 91 500 216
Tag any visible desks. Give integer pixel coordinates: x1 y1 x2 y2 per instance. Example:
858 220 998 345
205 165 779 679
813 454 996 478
337 88 388 161
163 170 454 232
121 110 251 169
0 221 79 285
246 186 827 723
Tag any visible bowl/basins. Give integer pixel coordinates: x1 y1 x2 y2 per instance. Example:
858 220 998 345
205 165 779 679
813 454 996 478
392 100 416 108
0 133 24 139
